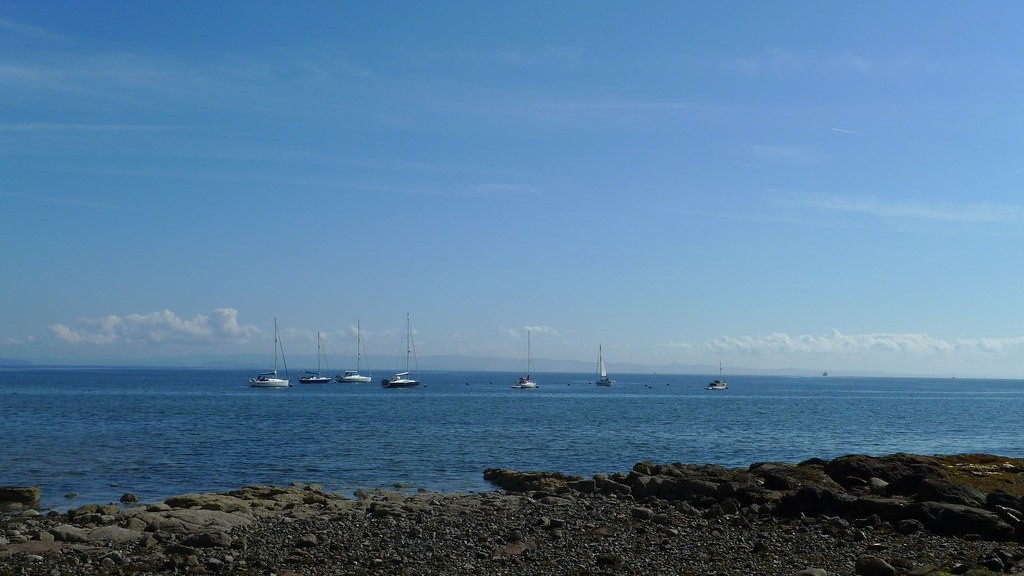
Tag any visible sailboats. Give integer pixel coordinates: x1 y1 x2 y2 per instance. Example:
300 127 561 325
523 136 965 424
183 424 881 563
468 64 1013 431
508 329 538 388
333 319 372 385
591 345 615 387
295 329 333 383
247 317 289 386
703 359 728 391
380 313 424 388
